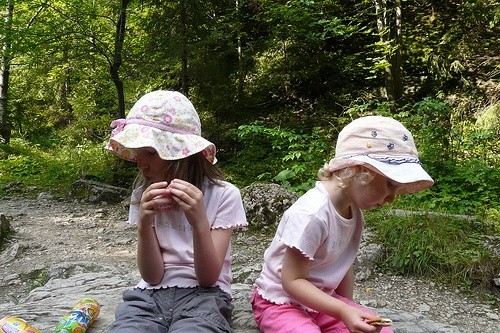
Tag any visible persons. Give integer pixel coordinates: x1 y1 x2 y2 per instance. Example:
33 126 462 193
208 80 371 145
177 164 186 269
250 115 436 333
103 89 249 333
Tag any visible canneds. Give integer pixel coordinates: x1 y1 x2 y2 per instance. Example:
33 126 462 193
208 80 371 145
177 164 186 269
0 315 42 333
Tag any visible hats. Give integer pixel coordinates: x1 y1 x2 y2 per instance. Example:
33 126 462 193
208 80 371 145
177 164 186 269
328 116 435 194
104 91 218 166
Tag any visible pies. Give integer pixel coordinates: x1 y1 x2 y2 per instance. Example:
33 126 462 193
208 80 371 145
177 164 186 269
368 317 393 327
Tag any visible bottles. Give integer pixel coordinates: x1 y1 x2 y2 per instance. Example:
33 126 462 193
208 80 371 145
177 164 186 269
52 298 100 333
0 315 43 333
